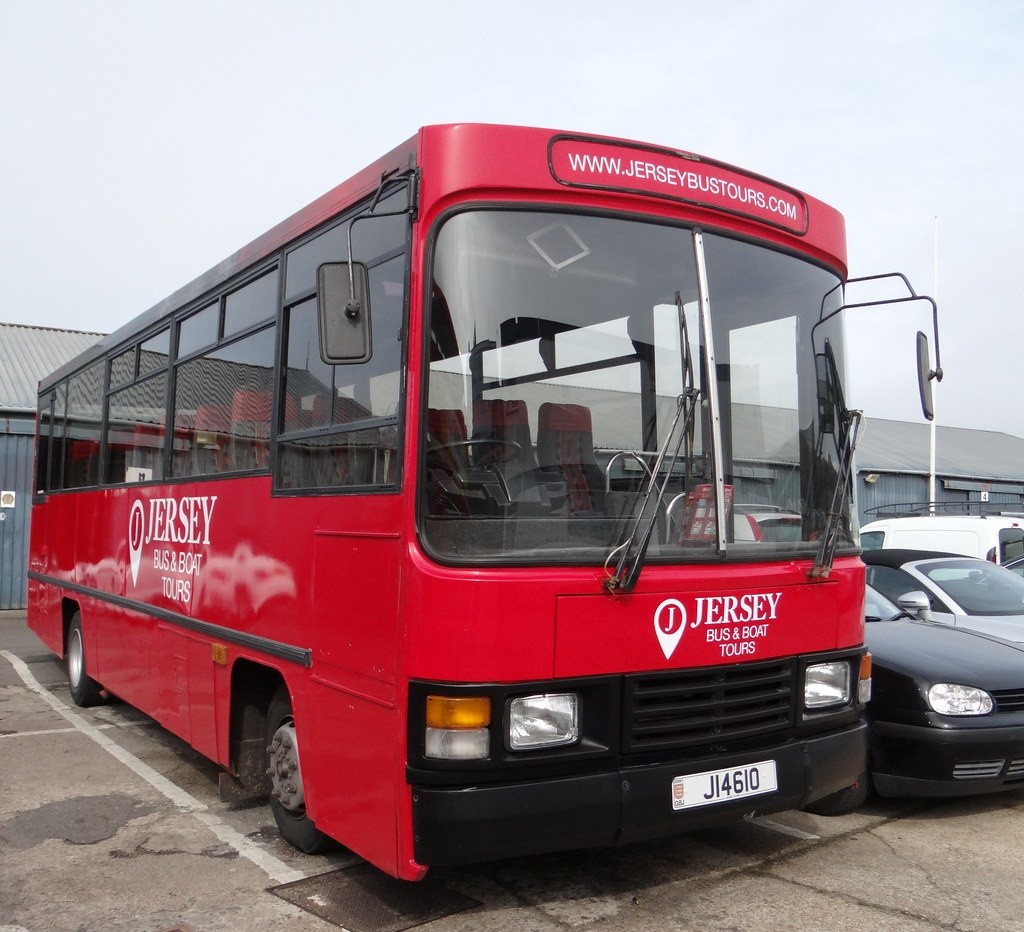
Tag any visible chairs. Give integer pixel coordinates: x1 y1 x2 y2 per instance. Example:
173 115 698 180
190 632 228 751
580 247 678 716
537 402 607 536
67 389 375 486
472 399 537 497
427 407 469 466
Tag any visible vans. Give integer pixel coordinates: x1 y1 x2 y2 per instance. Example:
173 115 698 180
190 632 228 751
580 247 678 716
859 515 1024 579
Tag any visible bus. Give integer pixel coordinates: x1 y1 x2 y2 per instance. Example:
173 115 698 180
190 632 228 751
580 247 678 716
25 125 943 881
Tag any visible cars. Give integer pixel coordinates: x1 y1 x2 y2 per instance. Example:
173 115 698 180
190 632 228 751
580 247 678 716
860 548 1024 644
799 582 1024 818
732 502 803 544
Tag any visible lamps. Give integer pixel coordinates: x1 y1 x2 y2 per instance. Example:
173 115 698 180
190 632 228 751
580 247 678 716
194 430 220 451
864 474 879 486
